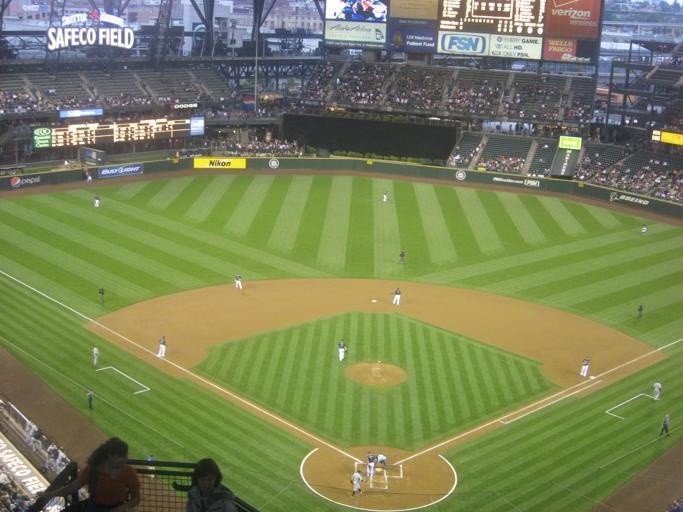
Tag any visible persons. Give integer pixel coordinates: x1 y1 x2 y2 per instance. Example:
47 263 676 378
653 382 661 400
37 437 141 512
636 304 642 323
338 340 345 361
235 273 242 289
186 458 238 512
0 469 34 512
392 288 401 305
94 347 98 366
1 54 683 208
87 391 93 408
159 336 166 356
351 450 388 494
580 359 589 377
671 498 683 512
25 416 70 474
660 414 671 436
